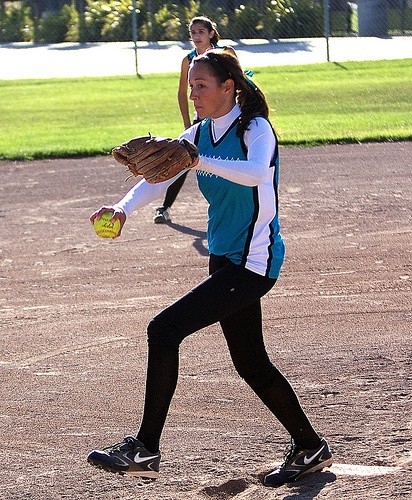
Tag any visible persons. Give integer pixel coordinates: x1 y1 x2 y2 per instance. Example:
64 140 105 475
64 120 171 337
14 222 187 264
153 16 239 224
87 49 334 487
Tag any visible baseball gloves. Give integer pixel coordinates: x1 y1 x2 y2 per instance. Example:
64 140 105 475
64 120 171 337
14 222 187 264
108 131 200 188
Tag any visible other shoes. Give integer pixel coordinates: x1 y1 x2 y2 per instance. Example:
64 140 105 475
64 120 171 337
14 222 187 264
153 207 171 223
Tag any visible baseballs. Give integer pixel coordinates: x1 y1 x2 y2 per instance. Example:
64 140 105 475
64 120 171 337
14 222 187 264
93 209 120 240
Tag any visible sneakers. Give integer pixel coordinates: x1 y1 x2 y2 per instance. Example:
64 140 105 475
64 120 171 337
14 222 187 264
263 439 332 488
87 436 160 479
